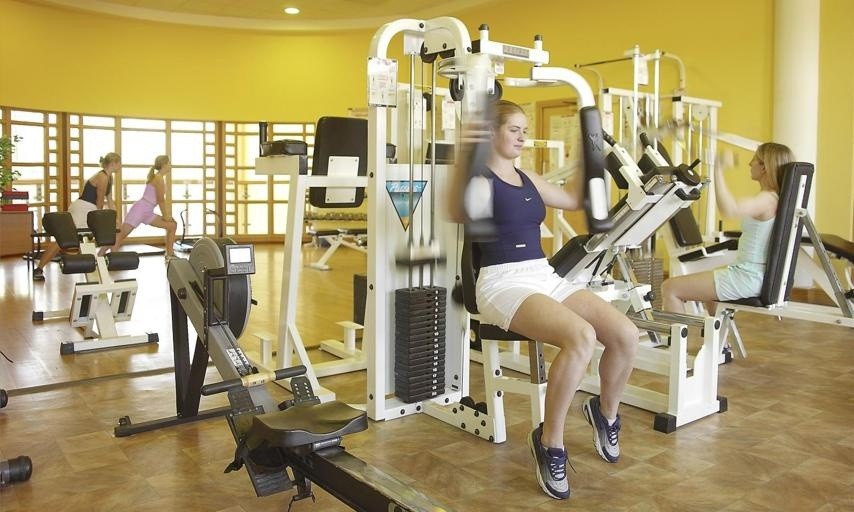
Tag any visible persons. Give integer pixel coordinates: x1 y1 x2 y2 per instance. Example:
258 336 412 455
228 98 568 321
96 155 180 262
441 99 639 500
661 142 797 353
33 152 121 282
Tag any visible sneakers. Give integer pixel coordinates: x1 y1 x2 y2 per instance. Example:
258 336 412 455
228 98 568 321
527 422 577 500
164 251 186 261
581 394 622 464
33 267 45 280
